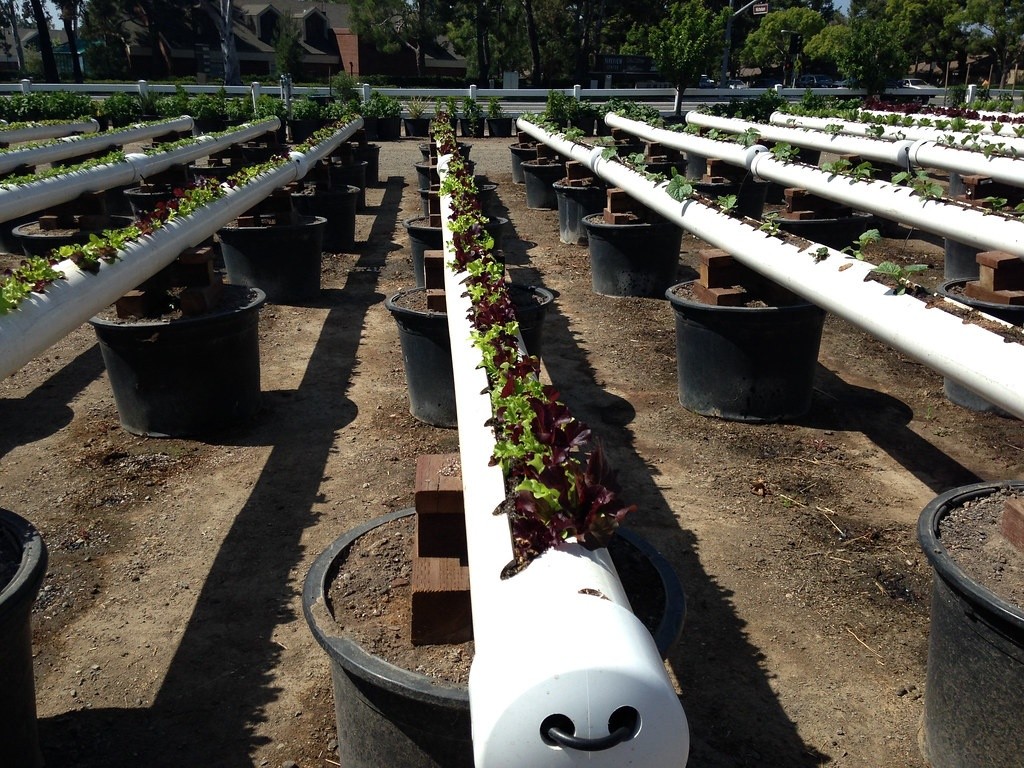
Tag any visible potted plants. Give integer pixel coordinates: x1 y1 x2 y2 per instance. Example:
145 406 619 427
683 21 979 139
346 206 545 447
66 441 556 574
71 80 614 138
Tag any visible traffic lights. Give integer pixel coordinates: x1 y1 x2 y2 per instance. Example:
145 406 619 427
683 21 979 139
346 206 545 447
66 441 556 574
784 62 792 73
793 32 803 53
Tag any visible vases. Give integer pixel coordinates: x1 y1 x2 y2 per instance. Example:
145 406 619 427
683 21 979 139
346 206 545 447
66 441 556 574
0 141 1024 768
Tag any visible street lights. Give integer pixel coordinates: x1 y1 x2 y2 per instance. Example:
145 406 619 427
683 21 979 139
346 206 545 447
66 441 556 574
781 29 800 88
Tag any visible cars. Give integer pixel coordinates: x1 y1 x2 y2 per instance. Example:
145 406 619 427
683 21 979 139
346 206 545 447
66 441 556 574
897 78 938 98
697 74 852 88
